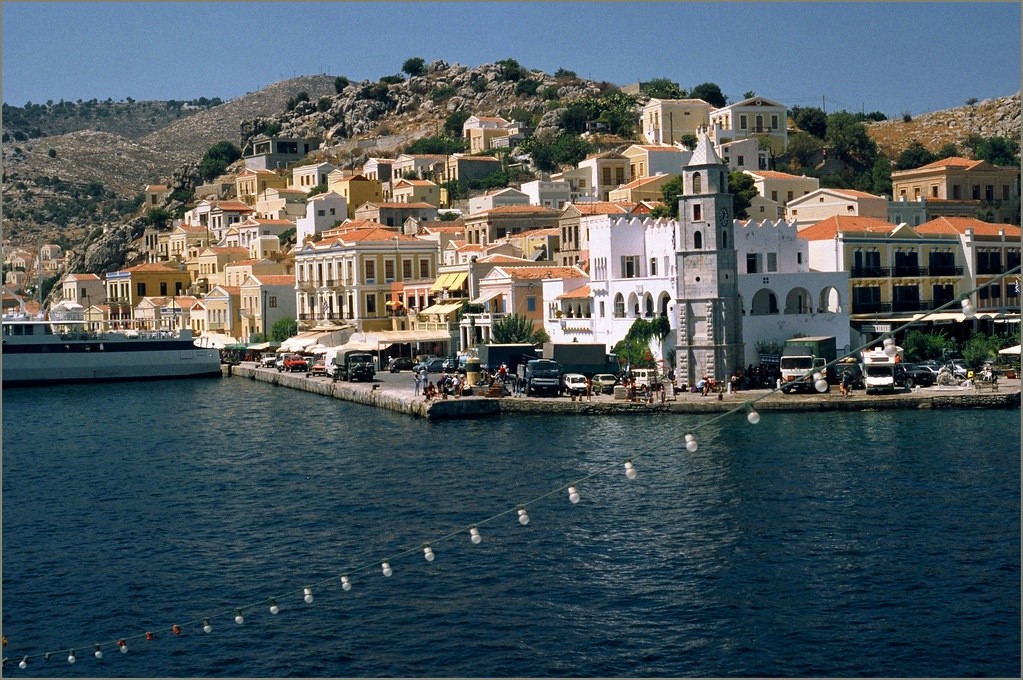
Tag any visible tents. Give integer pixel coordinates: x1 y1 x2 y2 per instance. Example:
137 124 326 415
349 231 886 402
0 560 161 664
370 328 453 371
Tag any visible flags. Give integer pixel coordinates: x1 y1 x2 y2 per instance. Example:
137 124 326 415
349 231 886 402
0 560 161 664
323 300 329 312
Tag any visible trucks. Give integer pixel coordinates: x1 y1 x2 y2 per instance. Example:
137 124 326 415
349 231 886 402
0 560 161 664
337 349 376 382
779 335 837 393
324 348 338 378
516 353 560 397
860 349 895 395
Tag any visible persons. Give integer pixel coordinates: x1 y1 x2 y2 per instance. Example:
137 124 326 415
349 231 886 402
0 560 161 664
413 374 421 396
420 366 430 391
428 381 438 395
840 372 849 398
437 374 467 394
584 377 593 400
695 377 710 397
661 383 665 404
895 354 901 366
731 375 738 394
513 373 522 398
628 383 653 402
496 362 511 385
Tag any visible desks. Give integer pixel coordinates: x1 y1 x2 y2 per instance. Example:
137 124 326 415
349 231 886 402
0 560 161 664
636 397 654 403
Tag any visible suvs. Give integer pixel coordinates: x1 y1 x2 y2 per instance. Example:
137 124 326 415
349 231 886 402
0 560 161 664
834 362 863 389
895 363 934 388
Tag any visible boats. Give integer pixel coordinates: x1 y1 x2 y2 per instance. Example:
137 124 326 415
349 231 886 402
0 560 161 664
0 313 223 384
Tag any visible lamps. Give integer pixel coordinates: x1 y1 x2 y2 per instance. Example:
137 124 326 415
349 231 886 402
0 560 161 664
95 646 102 658
568 483 580 503
813 368 827 392
744 401 760 424
960 292 976 317
880 332 896 356
118 641 128 653
304 589 313 603
468 526 481 544
684 430 697 452
624 458 636 479
382 563 392 577
423 544 435 562
202 620 212 633
517 506 529 525
235 610 243 623
68 650 76 663
340 574 351 590
19 655 29 669
270 599 279 614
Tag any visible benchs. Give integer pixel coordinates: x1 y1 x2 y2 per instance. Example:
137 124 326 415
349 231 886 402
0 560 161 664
974 381 999 393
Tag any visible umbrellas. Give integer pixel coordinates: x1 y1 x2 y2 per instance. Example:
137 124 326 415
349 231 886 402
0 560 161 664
384 300 404 311
276 344 334 357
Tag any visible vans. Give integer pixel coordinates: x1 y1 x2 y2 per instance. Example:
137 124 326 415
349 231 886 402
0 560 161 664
619 368 662 392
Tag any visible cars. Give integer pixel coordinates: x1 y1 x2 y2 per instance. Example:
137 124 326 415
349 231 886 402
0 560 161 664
917 365 939 377
312 360 326 377
940 364 976 379
562 373 590 396
386 355 473 373
591 374 624 394
259 352 314 373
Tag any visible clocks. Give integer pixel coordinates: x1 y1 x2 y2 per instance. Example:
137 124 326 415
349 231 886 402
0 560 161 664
719 207 729 227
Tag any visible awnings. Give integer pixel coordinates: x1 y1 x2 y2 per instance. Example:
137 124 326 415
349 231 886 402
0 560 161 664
419 302 463 315
280 336 318 349
224 340 277 351
429 273 468 290
